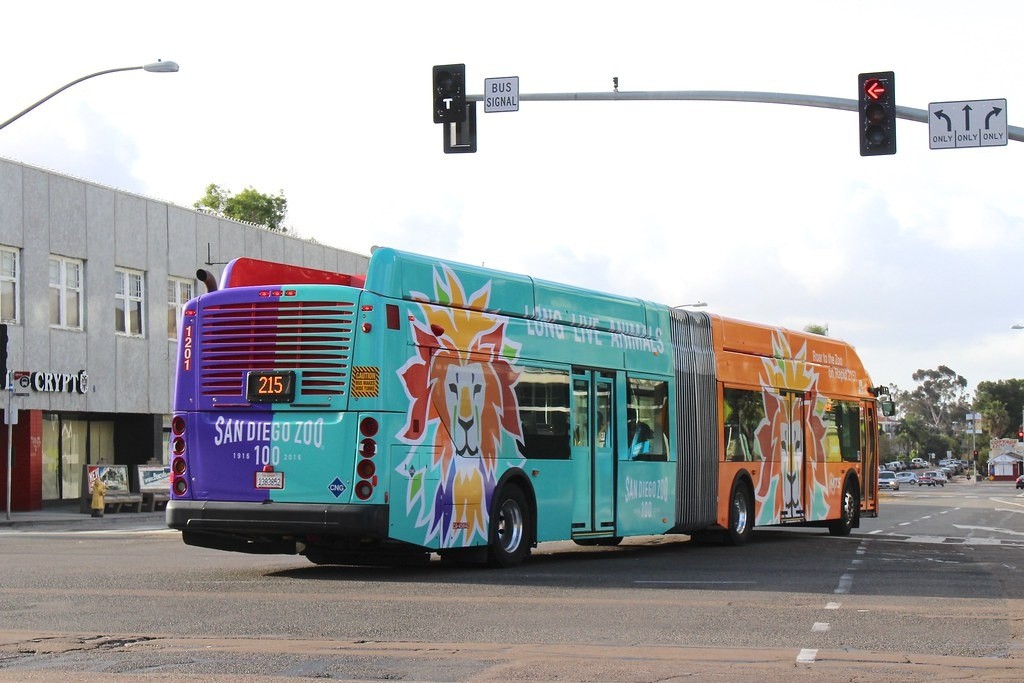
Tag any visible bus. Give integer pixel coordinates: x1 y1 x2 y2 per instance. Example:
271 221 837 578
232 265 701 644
164 244 896 571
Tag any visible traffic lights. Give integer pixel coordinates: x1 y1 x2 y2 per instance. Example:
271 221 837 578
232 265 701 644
1018 432 1023 442
432 63 467 124
973 450 978 461
858 71 897 157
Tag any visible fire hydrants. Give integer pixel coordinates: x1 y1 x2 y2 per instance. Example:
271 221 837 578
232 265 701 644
89 478 108 517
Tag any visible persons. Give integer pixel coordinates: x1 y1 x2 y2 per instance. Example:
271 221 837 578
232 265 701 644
91 473 107 518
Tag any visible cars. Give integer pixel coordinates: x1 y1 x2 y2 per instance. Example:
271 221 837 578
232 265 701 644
877 471 899 491
1015 474 1024 490
877 457 970 487
